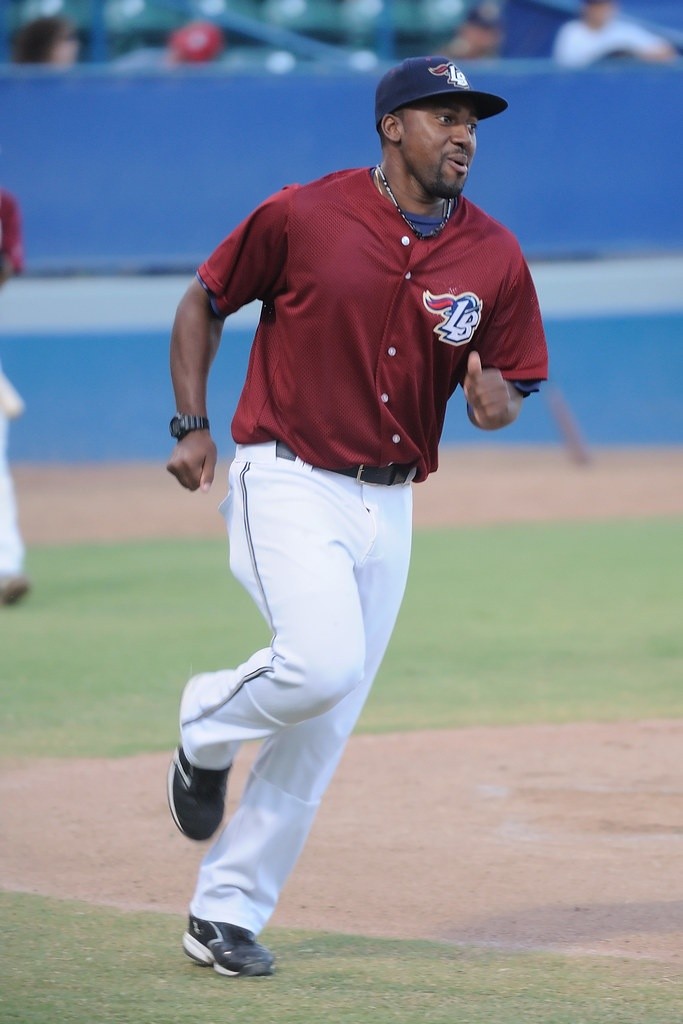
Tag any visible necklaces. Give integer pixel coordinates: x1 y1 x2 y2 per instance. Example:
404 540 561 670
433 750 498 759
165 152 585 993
372 163 452 237
374 169 447 232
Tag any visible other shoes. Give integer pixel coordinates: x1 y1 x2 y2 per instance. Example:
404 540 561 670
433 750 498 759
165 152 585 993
0 576 28 604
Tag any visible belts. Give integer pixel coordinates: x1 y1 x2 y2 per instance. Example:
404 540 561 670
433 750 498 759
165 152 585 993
276 440 419 487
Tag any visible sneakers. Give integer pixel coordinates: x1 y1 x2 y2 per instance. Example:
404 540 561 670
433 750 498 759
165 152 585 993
182 912 275 976
166 742 232 840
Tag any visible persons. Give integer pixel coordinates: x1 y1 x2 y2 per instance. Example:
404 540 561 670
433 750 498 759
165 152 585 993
163 58 548 975
0 0 683 603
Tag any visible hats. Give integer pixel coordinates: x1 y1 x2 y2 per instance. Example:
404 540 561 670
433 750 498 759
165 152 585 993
375 56 509 128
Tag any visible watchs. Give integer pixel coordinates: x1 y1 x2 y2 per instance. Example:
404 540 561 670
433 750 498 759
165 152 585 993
168 411 211 439
